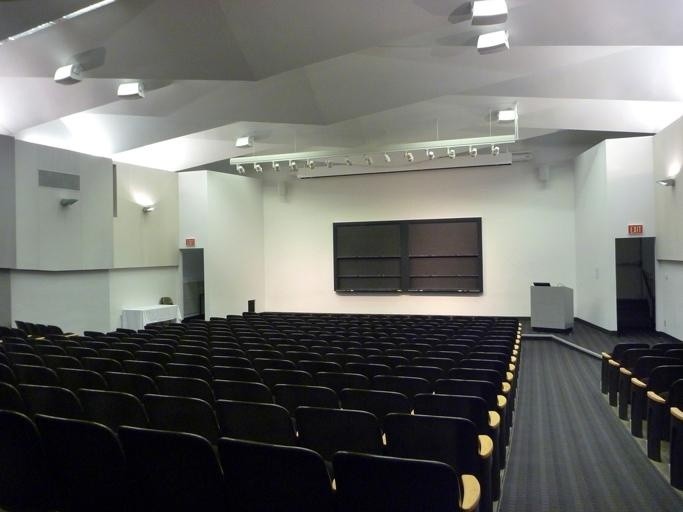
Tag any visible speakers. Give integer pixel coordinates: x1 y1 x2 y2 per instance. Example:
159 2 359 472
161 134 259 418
117 82 145 100
477 30 510 56
235 136 254 149
53 64 83 85
470 0 508 25
497 110 516 124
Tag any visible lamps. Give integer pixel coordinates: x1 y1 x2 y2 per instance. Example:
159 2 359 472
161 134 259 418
61 199 78 207
657 177 676 186
143 205 154 213
230 102 518 175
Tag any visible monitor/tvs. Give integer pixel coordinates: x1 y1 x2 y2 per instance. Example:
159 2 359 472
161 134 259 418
533 282 550 287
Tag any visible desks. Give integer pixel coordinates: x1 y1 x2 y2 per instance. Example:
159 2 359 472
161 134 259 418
122 304 184 334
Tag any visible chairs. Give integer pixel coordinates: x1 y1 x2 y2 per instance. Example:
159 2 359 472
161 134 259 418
161 297 174 324
602 342 683 492
